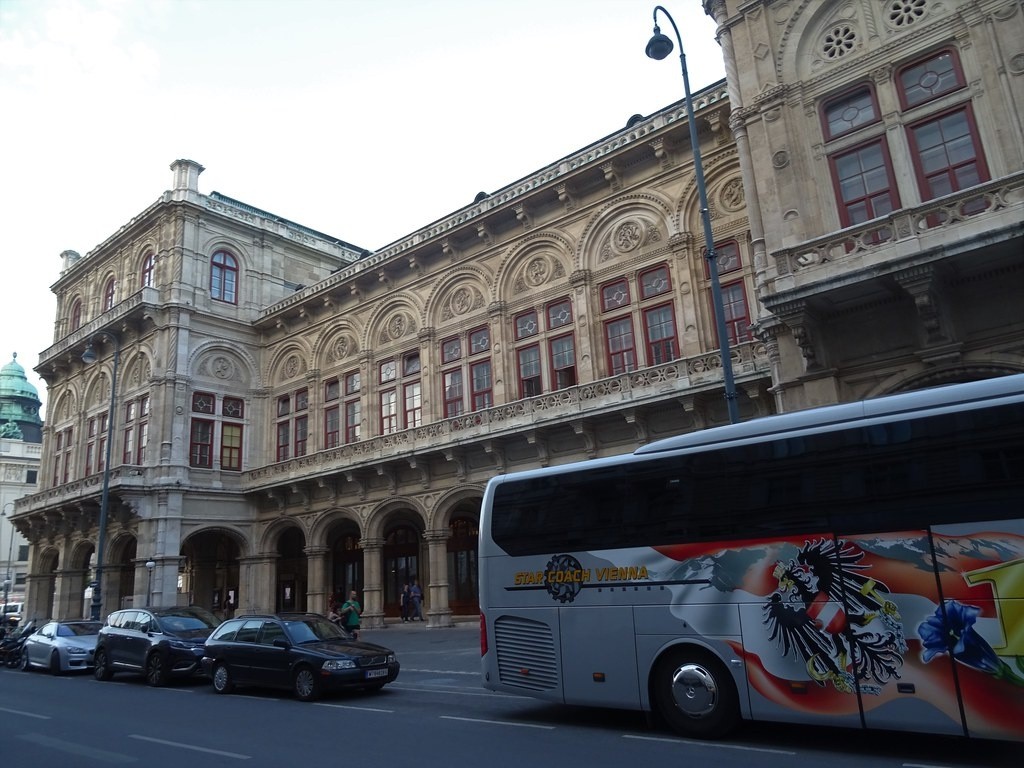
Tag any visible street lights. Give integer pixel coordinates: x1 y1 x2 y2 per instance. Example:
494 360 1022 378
0 502 17 613
644 4 739 426
82 330 121 622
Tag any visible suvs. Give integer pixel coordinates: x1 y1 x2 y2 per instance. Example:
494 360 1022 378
202 612 402 702
94 605 223 690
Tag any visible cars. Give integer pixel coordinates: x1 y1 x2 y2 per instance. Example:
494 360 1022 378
0 603 22 620
19 619 104 676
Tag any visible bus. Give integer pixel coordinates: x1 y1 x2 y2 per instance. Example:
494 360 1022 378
478 372 1024 743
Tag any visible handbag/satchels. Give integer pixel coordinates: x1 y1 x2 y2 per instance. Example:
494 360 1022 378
341 601 350 626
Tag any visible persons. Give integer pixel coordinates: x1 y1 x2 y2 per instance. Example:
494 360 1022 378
322 591 362 641
400 580 425 622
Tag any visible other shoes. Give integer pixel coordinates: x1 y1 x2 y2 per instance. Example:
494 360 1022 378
421 619 424 621
410 618 414 621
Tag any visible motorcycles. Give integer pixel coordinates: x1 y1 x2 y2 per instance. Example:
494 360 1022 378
0 617 36 669
